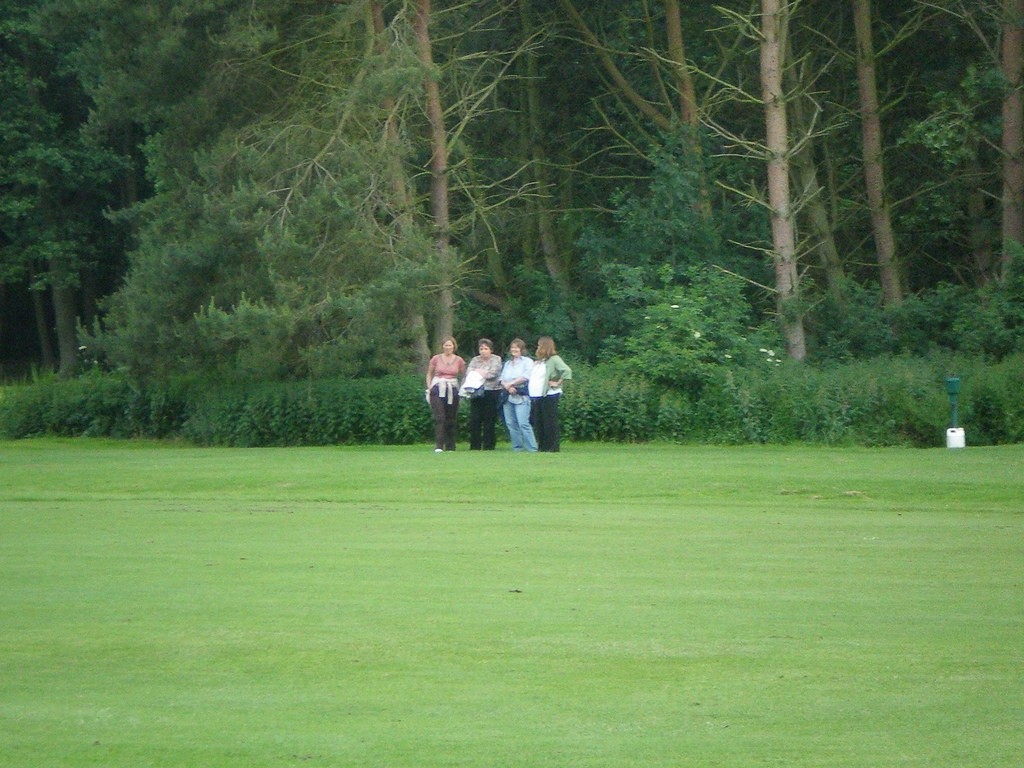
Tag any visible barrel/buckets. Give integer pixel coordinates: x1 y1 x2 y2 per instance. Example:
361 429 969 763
946 428 965 448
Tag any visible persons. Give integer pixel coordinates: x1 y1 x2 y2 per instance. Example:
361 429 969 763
528 336 572 452
463 338 508 451
425 335 466 453
499 339 538 452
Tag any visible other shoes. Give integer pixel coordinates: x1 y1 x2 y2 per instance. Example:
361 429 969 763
435 449 443 452
446 450 454 452
